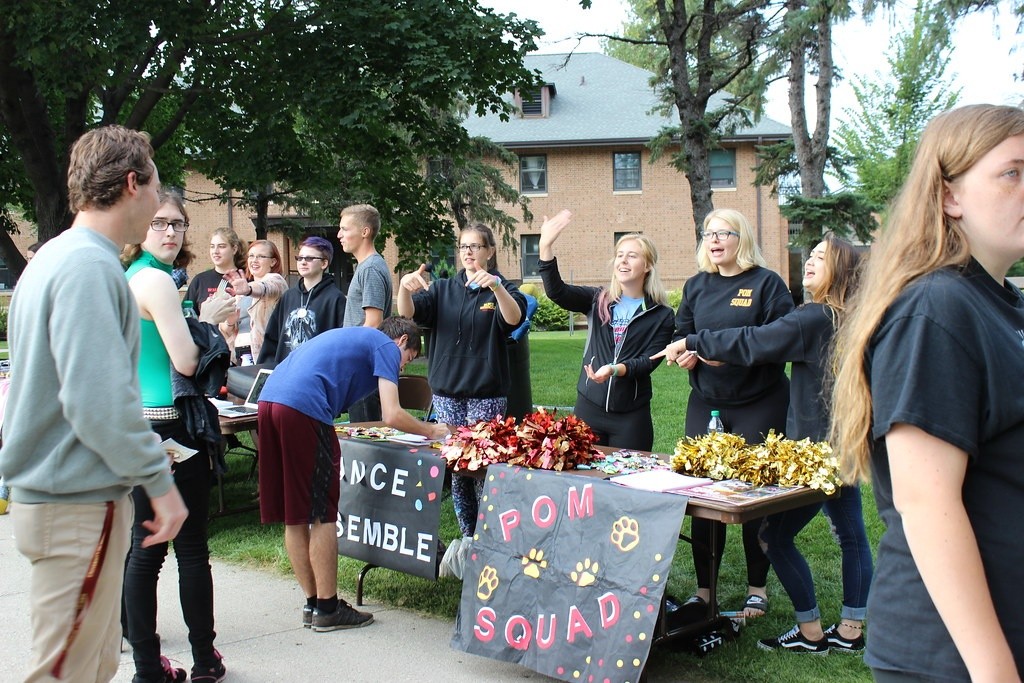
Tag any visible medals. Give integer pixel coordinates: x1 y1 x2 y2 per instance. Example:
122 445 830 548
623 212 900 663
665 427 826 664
298 309 307 318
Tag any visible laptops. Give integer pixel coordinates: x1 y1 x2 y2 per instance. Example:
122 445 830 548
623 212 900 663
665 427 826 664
217 369 273 418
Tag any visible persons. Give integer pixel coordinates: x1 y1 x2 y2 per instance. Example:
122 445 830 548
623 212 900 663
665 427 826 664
0 125 188 683
829 104 1024 683
256 236 346 369
122 186 240 683
397 222 527 538
538 209 675 455
255 315 451 632
337 203 392 420
183 227 289 364
649 236 873 656
672 208 791 615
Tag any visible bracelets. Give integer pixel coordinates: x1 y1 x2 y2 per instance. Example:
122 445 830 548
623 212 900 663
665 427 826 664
610 366 618 376
225 320 235 326
489 276 501 291
245 286 253 296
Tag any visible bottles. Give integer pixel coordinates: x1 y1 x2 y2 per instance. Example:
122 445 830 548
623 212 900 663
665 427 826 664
707 410 723 434
183 300 198 321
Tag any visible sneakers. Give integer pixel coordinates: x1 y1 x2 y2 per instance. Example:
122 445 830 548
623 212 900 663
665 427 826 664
311 599 374 632
132 655 187 683
824 622 866 653
757 623 830 655
191 649 227 683
302 605 315 628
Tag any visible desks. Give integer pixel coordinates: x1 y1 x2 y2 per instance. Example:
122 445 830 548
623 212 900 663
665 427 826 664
334 420 840 666
204 393 258 514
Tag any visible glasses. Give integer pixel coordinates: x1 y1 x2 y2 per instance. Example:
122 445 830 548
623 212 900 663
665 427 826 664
247 255 274 260
295 255 325 262
457 243 487 252
150 220 189 232
700 231 741 241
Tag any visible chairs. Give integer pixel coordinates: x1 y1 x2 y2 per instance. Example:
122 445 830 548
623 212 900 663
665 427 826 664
401 373 434 421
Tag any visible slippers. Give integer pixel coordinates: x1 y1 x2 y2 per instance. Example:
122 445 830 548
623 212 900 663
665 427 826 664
741 593 768 617
684 595 721 609
